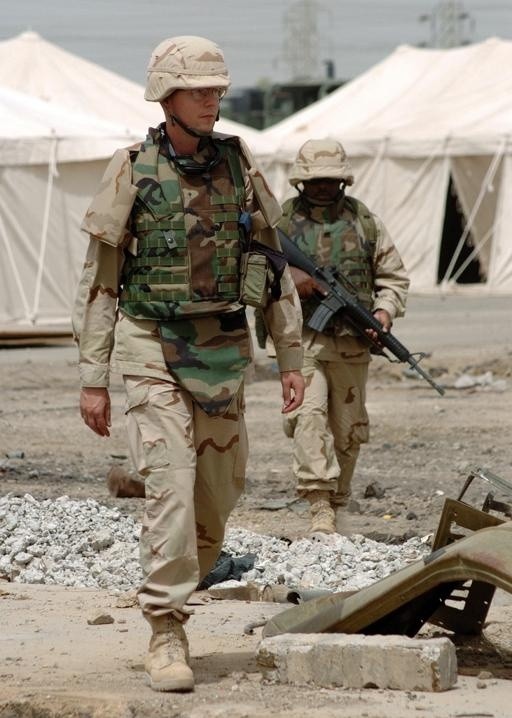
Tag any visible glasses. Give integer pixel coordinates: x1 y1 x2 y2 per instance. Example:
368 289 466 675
167 152 223 175
179 88 226 101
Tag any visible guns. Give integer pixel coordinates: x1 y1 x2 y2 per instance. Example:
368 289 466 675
274 229 447 399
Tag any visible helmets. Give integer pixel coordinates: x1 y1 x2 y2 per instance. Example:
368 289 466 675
288 138 354 186
144 36 232 102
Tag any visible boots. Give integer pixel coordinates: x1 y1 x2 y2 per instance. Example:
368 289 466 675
143 612 195 692
306 488 335 534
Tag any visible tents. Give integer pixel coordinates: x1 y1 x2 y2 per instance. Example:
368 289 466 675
253 36 512 295
0 30 259 347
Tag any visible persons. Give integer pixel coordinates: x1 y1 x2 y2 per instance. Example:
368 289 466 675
71 36 306 693
254 137 410 534
